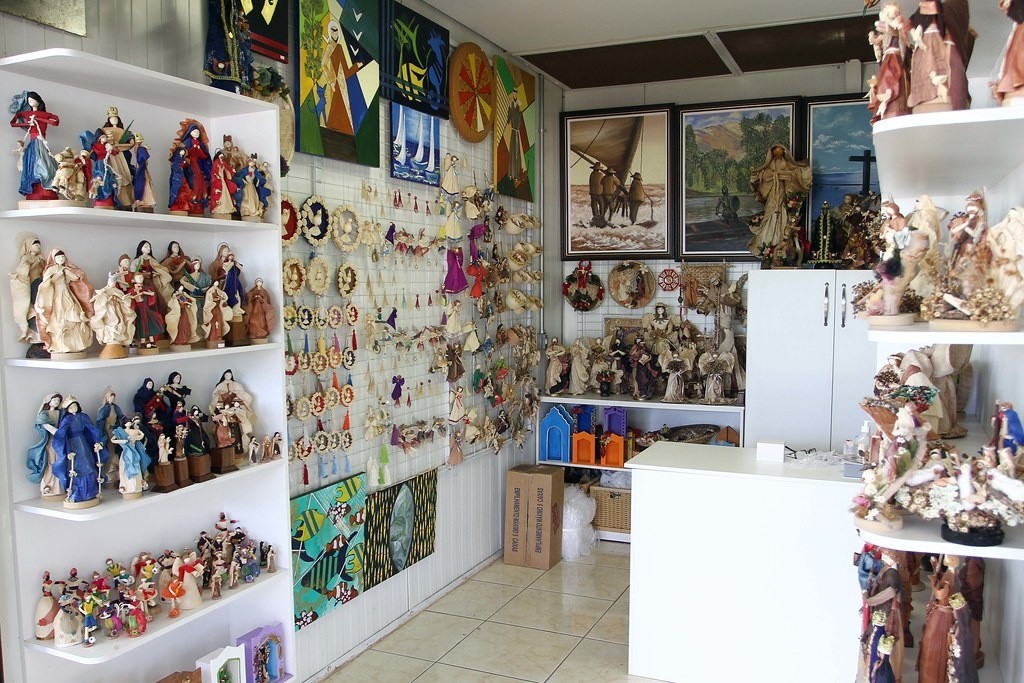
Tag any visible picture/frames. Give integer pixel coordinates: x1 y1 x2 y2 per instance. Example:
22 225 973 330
493 55 536 203
559 102 676 261
233 0 289 64
675 95 803 262
803 92 882 264
389 100 441 188
293 0 381 169
380 0 449 121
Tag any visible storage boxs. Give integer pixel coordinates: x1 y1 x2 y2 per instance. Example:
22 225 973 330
503 464 565 571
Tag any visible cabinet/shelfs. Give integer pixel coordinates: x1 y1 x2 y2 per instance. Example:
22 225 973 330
857 105 1024 683
536 393 746 545
744 270 880 455
0 48 298 683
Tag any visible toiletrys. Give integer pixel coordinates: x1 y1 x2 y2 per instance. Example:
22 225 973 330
855 420 874 464
